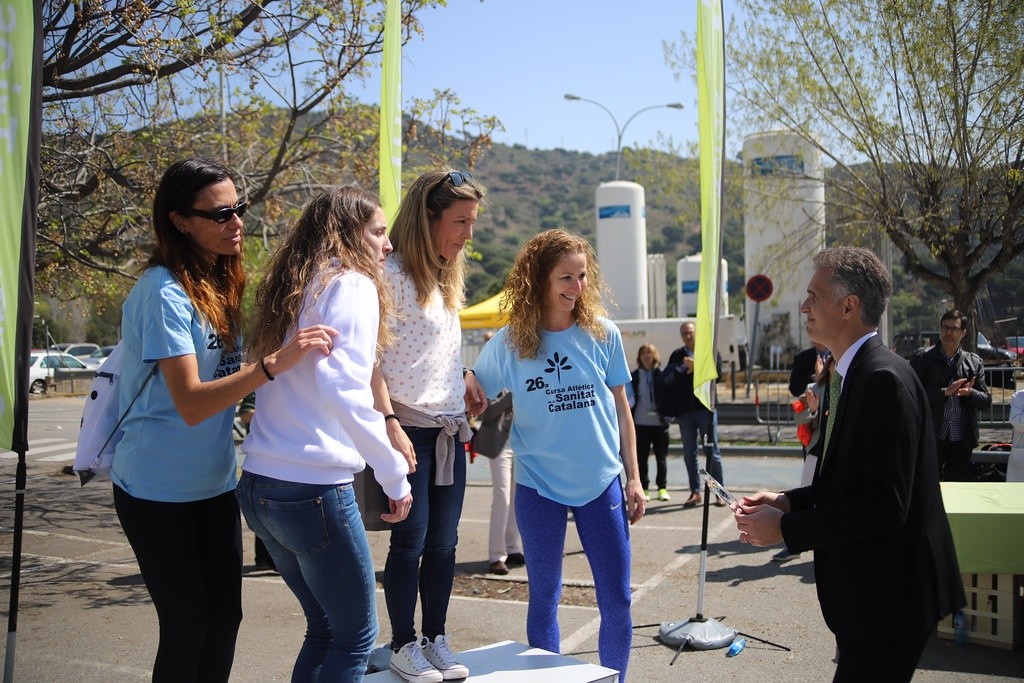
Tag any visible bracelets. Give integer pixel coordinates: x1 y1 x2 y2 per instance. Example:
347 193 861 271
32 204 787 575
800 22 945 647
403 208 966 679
385 414 399 422
261 357 275 381
463 367 477 379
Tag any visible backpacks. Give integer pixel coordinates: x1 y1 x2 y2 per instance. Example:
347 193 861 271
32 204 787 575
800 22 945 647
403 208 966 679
72 263 196 488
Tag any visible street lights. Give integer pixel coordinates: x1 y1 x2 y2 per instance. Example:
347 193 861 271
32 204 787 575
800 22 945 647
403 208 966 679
562 93 685 179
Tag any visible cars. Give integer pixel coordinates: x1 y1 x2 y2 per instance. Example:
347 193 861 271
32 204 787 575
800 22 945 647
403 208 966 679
89 346 116 362
891 329 1024 372
29 352 98 396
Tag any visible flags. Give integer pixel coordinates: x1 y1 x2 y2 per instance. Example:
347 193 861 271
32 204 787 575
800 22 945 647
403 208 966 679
379 0 404 239
692 0 726 409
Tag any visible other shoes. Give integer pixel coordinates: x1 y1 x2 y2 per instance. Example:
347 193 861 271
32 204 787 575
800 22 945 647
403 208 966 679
508 553 525 564
659 488 669 501
717 495 726 507
773 545 800 561
491 560 508 573
257 559 272 570
645 488 651 502
684 493 701 507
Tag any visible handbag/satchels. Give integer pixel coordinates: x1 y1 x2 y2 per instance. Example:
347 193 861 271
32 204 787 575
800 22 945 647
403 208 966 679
794 401 812 446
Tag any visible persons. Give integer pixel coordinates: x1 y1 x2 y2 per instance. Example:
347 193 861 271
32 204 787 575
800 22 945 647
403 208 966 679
663 322 729 506
110 158 339 683
910 309 991 482
628 343 671 501
475 228 646 683
730 245 969 683
374 170 488 682
794 356 838 459
488 438 525 574
1007 390 1024 482
236 183 418 683
789 341 834 397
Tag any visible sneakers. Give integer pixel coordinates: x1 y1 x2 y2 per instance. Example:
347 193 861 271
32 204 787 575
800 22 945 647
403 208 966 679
423 634 469 679
389 641 444 683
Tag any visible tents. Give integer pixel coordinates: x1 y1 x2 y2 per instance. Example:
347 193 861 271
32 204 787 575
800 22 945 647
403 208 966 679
459 286 519 330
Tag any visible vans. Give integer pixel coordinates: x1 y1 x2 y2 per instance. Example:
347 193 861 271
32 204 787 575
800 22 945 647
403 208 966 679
51 342 102 359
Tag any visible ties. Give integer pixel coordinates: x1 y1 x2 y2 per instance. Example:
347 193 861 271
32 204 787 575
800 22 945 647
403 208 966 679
821 353 827 365
819 368 842 476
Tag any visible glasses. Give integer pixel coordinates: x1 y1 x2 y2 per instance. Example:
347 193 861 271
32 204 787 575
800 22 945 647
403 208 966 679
942 325 962 334
429 172 472 196
186 200 247 224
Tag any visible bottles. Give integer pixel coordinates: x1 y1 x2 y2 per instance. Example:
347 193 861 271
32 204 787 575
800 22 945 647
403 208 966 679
728 637 745 656
